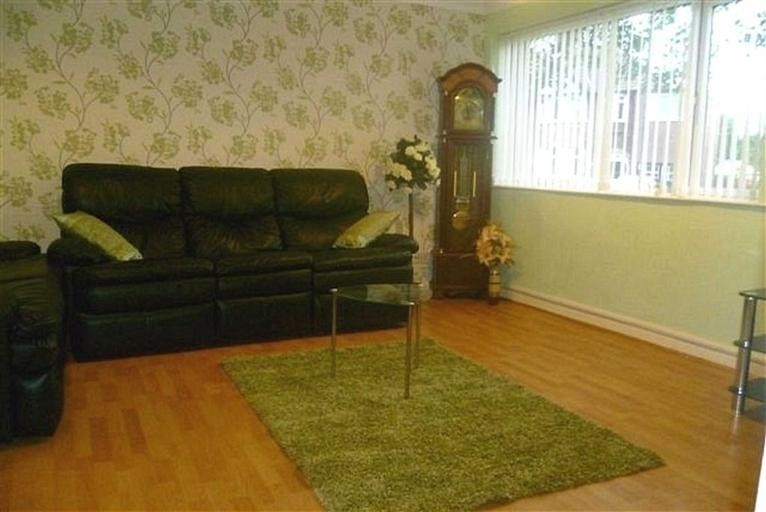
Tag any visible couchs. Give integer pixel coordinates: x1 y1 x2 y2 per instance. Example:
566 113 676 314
46 162 420 364
1 240 68 444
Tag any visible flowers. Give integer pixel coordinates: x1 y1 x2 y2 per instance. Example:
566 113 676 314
386 134 443 237
458 219 516 272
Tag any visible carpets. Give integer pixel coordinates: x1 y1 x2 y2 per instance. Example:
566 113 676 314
222 335 667 512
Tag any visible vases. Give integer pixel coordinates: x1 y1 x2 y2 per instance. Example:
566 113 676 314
487 268 502 305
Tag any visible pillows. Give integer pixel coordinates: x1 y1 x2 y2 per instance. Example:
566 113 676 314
331 209 402 249
51 209 145 263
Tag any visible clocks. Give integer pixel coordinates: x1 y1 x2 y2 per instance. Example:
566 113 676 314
430 62 502 300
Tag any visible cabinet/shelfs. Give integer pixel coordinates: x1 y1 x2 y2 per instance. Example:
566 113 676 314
726 288 766 415
